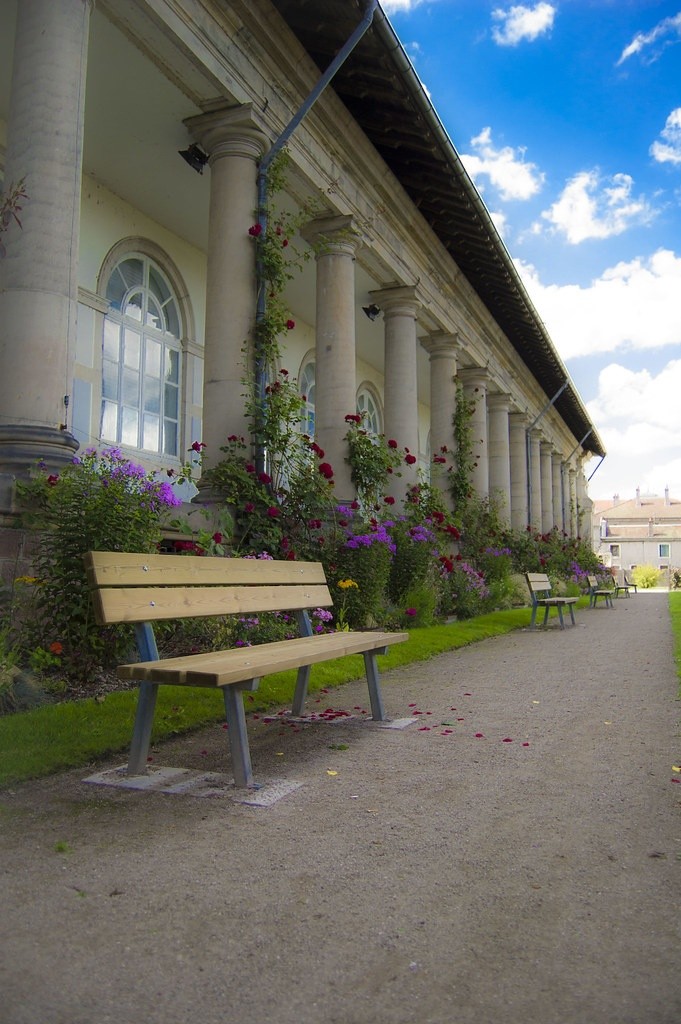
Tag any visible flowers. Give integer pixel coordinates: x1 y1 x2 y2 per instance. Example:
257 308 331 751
12 214 626 661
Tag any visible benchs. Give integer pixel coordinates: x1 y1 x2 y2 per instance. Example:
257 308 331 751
79 549 410 790
521 572 577 631
585 576 614 608
623 577 637 595
611 577 631 599
